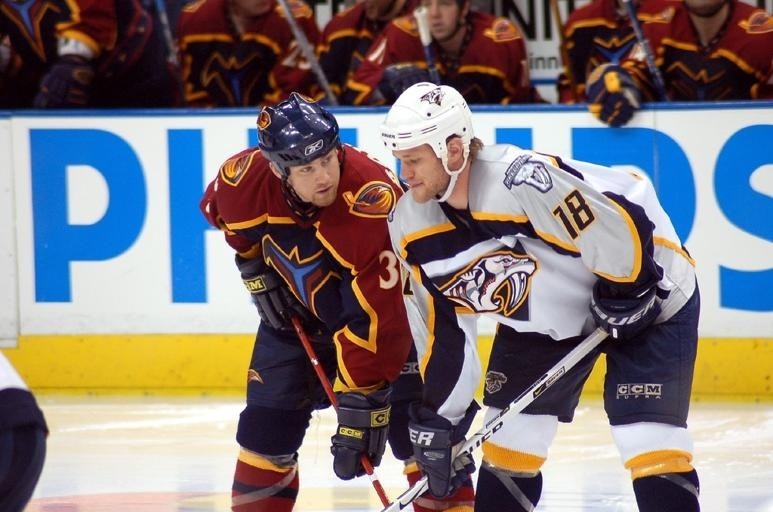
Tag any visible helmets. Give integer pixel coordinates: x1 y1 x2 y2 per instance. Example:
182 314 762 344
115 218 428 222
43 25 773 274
380 80 477 158
255 89 341 177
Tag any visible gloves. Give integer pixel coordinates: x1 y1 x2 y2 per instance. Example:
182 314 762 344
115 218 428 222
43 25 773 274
326 384 398 479
580 279 669 356
584 56 643 129
231 251 308 331
30 53 106 108
376 59 447 106
406 392 478 498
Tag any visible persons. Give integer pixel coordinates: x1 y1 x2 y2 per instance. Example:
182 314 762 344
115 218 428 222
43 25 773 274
198 91 475 512
0 352 49 512
0 0 773 127
382 82 700 512
